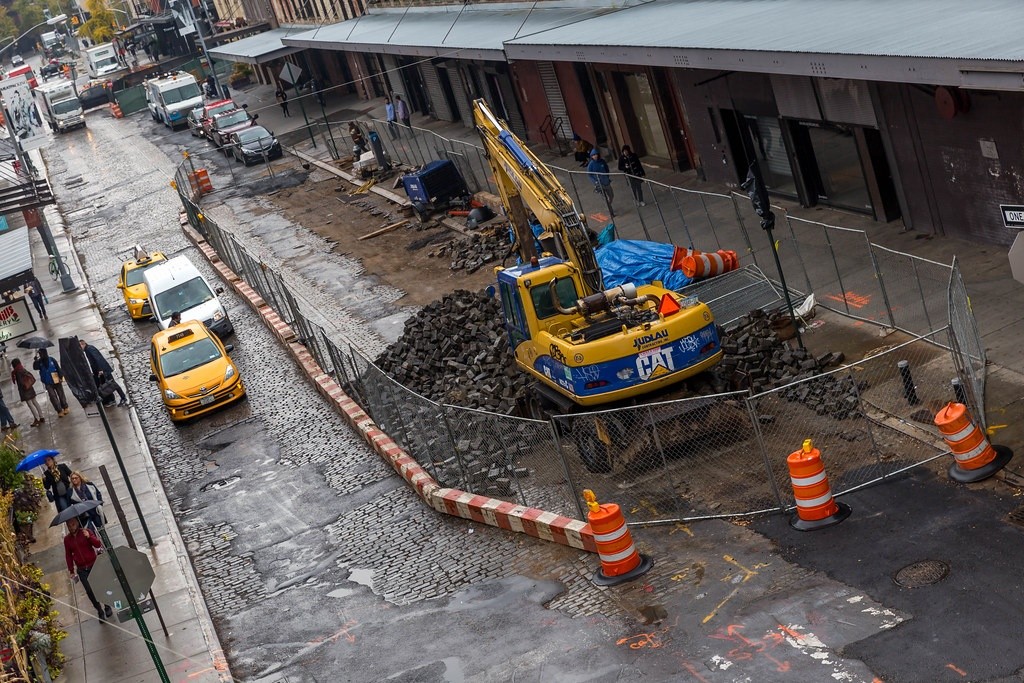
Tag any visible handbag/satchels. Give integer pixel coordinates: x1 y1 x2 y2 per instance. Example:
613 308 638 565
45 297 49 304
20 371 36 390
593 188 605 196
98 373 118 399
275 91 283 103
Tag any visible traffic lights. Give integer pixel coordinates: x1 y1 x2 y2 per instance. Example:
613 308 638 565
71 17 79 25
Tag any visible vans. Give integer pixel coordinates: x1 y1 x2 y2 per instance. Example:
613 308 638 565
145 254 236 336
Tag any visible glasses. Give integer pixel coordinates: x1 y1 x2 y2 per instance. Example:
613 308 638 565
72 477 79 480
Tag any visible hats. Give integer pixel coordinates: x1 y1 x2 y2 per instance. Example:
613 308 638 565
590 148 600 156
571 133 581 141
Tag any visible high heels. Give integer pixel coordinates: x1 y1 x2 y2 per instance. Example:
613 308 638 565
30 419 40 426
40 418 45 423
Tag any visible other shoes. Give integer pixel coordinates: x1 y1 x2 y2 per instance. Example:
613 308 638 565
639 201 646 206
59 411 64 417
634 200 639 206
583 161 589 167
64 407 69 414
578 162 585 167
10 424 20 429
40 313 43 318
105 606 112 618
98 611 105 624
1 426 10 431
44 313 49 319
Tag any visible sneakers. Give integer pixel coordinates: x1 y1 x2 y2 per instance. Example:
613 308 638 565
104 400 116 408
118 398 128 407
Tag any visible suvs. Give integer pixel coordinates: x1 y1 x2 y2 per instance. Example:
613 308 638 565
211 106 260 157
202 99 248 141
76 81 111 112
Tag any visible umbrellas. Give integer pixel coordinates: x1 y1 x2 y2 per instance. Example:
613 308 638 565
16 449 59 472
48 500 105 528
16 336 55 356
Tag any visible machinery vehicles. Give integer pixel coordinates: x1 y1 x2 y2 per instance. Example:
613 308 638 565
468 98 753 469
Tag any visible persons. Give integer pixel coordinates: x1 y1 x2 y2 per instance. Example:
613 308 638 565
130 48 137 60
348 121 370 161
202 74 218 99
79 340 128 407
24 277 48 320
68 471 108 554
395 95 415 139
11 358 45 426
63 518 113 624
753 124 773 160
617 145 646 206
384 98 401 140
143 41 159 62
33 348 70 417
276 87 290 117
0 389 20 432
43 455 72 513
587 149 614 212
571 133 593 167
168 312 181 328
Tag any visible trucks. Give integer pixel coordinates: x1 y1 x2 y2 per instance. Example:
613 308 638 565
143 69 206 128
36 79 90 131
4 64 40 97
40 31 59 49
83 42 129 79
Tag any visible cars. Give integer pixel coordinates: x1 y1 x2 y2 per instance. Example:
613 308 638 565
188 106 209 138
229 125 283 166
145 319 251 422
41 59 78 78
11 54 25 66
117 245 170 322
